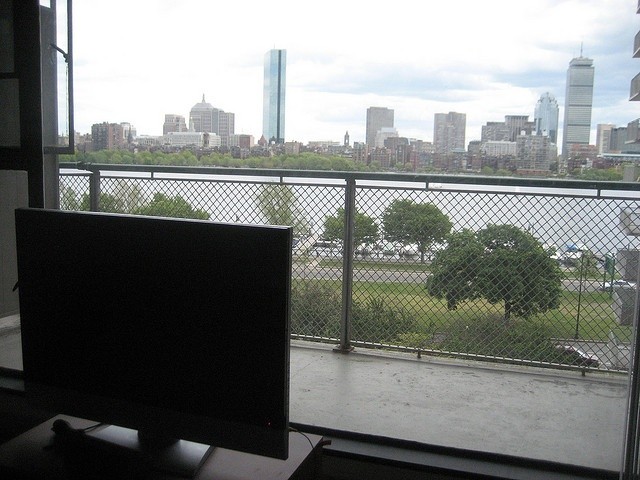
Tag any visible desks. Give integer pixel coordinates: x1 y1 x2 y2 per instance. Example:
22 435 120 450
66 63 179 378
0 412 324 480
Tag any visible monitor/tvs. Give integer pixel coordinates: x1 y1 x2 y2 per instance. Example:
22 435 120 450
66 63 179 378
15 208 293 479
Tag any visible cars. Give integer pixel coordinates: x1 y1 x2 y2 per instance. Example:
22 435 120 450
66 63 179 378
556 345 600 367
599 279 637 292
